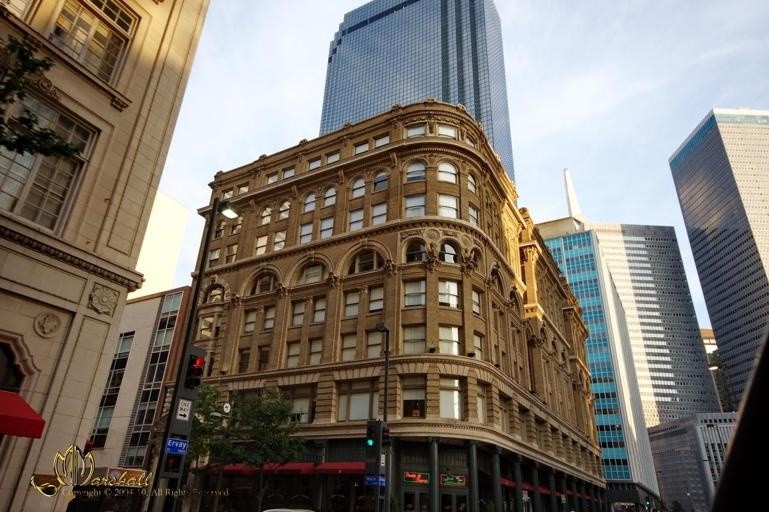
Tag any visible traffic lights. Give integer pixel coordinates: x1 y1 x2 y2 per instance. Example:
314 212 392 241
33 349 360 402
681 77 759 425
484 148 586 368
184 353 203 389
366 422 379 457
163 454 183 472
644 496 651 509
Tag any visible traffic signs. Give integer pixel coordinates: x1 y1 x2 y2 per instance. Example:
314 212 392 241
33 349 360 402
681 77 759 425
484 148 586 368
165 438 189 453
175 399 192 422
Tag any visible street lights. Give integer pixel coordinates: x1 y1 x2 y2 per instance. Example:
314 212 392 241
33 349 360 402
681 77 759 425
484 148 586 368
210 410 232 511
373 320 390 510
143 195 240 510
